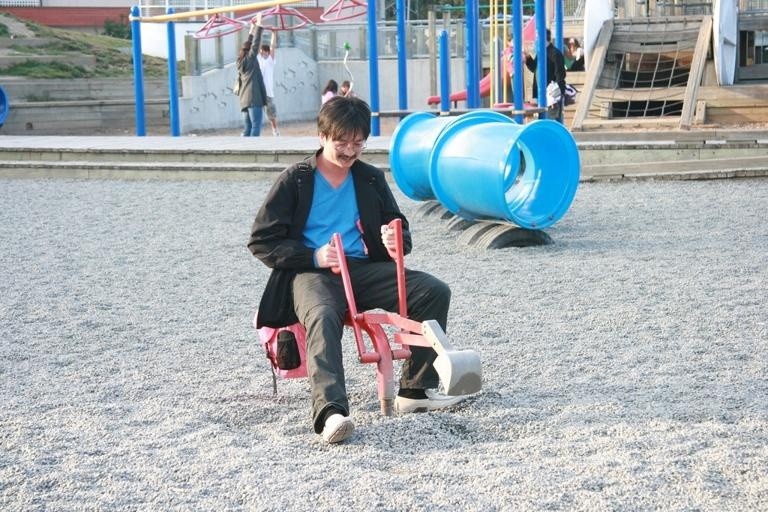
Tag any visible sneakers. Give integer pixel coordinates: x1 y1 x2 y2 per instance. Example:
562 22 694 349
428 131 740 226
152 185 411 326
322 414 354 442
394 389 465 412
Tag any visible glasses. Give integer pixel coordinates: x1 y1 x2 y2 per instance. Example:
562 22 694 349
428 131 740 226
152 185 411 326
333 141 367 151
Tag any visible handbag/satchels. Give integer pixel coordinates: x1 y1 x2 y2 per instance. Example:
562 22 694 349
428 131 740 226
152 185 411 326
255 313 308 377
234 74 240 96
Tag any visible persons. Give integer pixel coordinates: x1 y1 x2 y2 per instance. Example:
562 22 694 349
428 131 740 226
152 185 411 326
569 36 584 61
321 80 339 105
248 96 463 443
563 38 584 71
522 28 566 124
339 81 357 98
256 27 280 137
236 13 268 136
